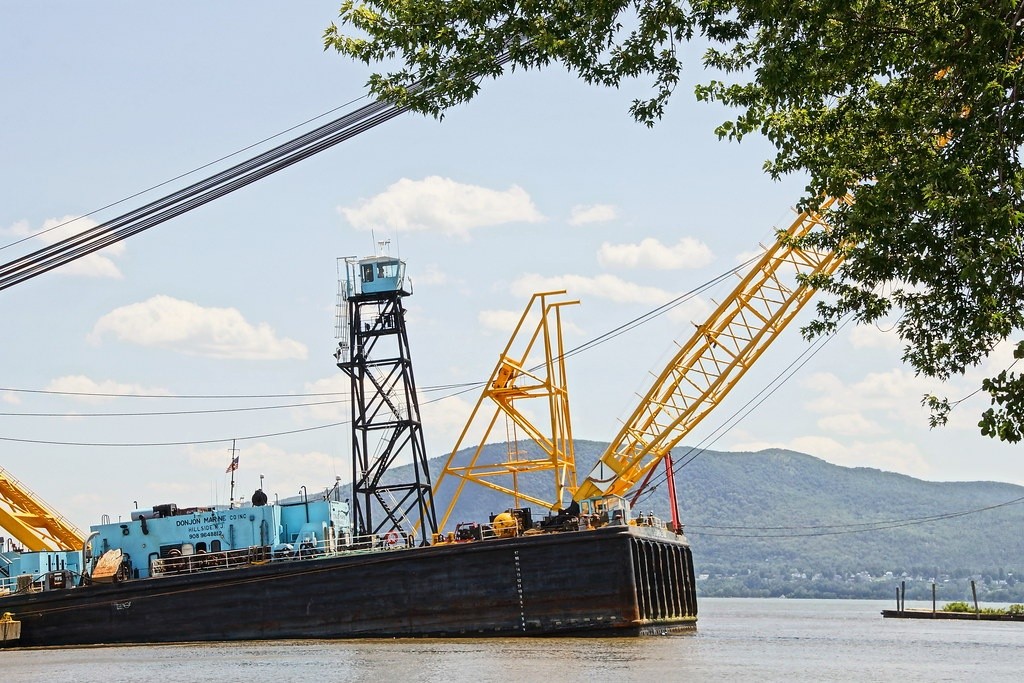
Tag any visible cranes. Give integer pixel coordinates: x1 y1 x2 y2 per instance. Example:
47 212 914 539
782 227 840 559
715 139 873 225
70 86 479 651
413 46 972 545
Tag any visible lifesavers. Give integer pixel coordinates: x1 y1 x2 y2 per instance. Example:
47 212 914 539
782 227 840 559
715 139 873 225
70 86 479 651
384 530 398 544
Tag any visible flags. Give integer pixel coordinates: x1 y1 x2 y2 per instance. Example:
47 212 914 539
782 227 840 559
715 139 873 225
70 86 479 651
226 456 238 473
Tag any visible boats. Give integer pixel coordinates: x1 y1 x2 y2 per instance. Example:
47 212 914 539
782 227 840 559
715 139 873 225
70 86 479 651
0 229 699 649
